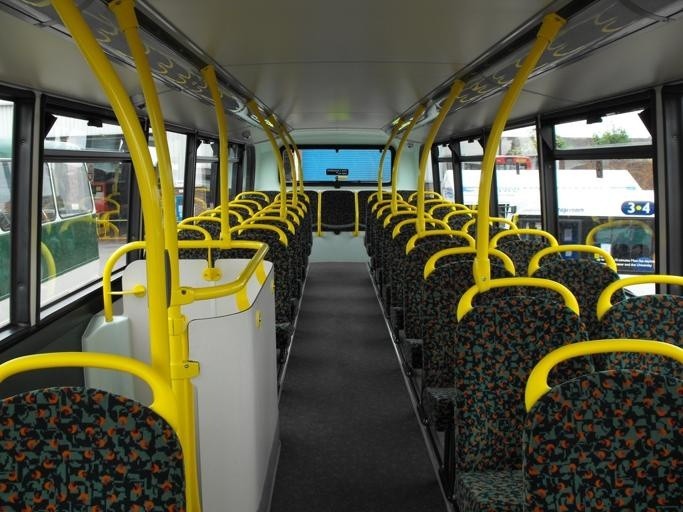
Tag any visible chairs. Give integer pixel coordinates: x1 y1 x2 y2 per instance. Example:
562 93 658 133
0 355 191 511
360 186 683 508
167 188 319 381
322 187 356 235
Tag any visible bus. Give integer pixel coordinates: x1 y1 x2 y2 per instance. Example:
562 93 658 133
0 139 103 334
439 166 641 226
499 186 655 299
7 123 224 282
